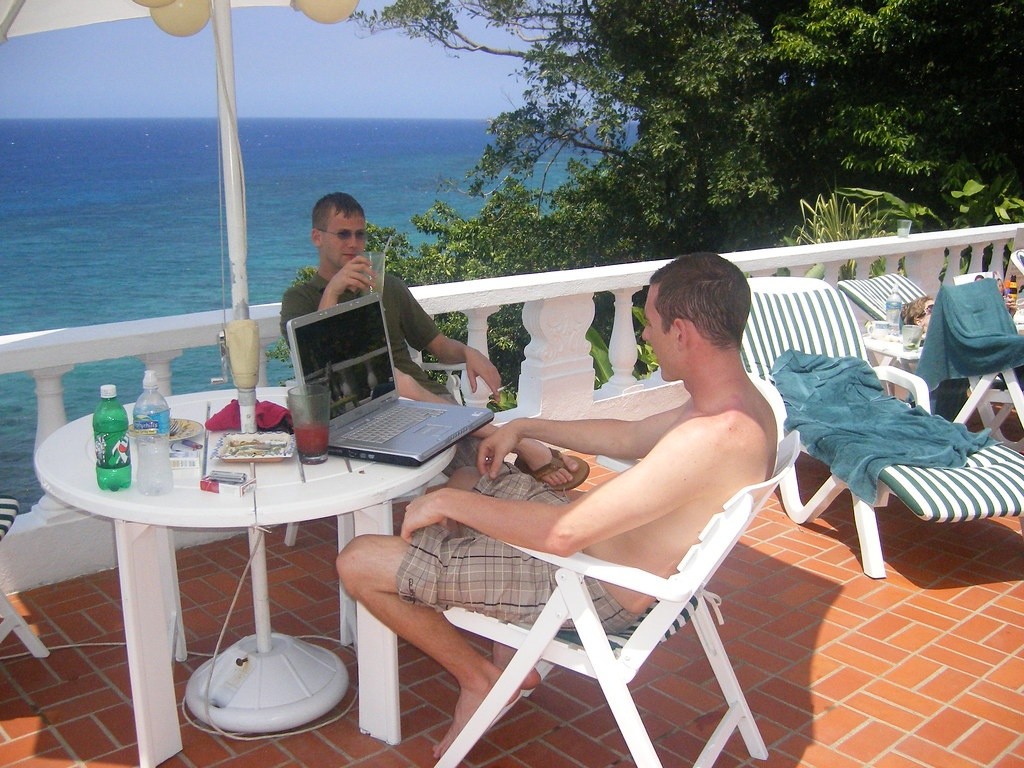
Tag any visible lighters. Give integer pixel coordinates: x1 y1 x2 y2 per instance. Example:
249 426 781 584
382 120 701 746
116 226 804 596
182 440 202 449
210 471 247 482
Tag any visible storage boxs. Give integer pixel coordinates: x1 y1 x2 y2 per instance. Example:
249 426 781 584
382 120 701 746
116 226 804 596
201 477 254 497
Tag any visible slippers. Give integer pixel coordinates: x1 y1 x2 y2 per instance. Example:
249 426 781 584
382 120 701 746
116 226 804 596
515 444 590 491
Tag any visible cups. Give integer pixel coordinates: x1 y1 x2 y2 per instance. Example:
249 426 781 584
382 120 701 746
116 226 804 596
902 325 923 352
896 220 912 237
288 385 332 465
867 321 890 340
1016 298 1024 316
358 250 384 296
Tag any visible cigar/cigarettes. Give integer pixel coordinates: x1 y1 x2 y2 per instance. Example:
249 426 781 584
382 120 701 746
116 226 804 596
497 386 506 392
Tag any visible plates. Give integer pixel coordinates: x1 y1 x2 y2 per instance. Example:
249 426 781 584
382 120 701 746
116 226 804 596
210 432 295 462
127 419 204 441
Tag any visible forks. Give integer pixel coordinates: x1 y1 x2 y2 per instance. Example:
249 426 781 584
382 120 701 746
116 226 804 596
171 422 179 434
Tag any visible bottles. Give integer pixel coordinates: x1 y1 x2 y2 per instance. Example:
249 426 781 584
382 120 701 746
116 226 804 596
92 384 132 491
886 287 902 336
133 370 173 497
1008 275 1017 303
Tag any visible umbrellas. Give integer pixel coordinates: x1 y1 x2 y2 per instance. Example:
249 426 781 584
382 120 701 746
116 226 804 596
0 0 364 650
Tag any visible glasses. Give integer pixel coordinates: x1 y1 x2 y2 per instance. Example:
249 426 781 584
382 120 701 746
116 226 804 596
315 225 368 242
919 304 934 319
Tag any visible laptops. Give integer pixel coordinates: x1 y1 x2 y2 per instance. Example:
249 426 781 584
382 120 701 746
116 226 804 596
286 294 495 467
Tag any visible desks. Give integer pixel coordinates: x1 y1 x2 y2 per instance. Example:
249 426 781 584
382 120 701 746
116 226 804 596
33 386 456 768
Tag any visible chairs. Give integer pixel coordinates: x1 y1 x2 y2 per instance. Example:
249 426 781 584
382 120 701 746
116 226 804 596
0 495 50 658
285 342 472 648
432 430 801 768
738 248 1024 579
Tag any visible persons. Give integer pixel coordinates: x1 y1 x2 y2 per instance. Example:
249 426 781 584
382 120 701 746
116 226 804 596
279 191 590 494
335 251 777 758
902 293 1018 341
975 275 984 281
997 278 1004 295
